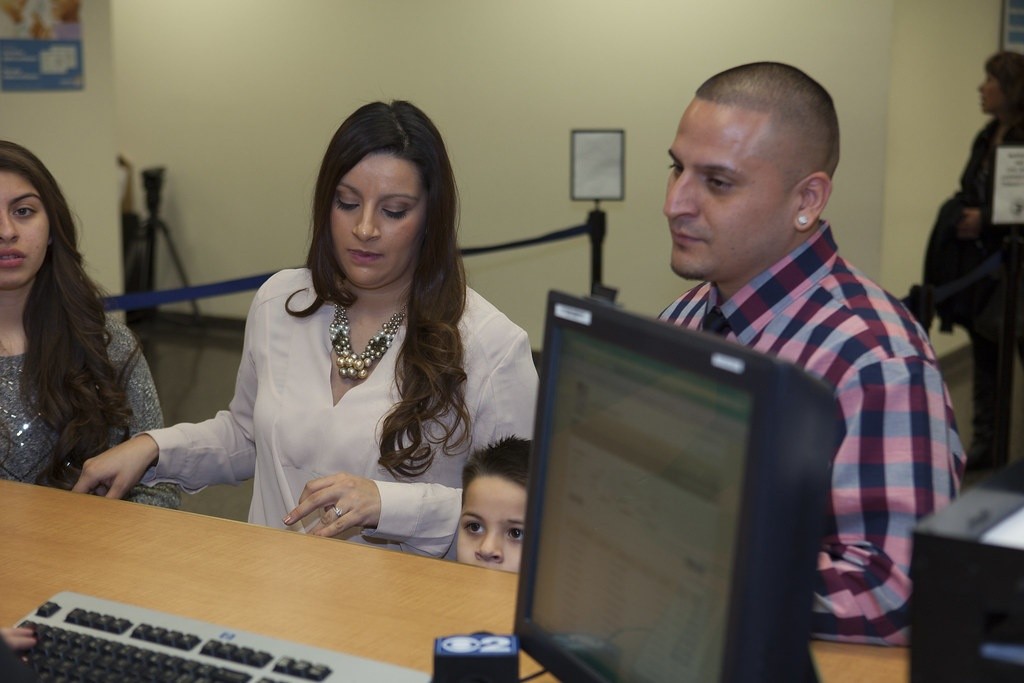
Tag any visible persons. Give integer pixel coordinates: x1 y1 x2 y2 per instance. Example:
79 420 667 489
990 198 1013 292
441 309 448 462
0 139 182 510
71 99 538 560
457 434 534 575
960 50 1024 473
655 62 970 648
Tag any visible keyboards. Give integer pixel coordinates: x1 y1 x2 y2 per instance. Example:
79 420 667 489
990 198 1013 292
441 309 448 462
8 591 433 683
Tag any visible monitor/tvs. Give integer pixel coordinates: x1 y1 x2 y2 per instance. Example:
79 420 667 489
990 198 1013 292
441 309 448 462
514 287 848 683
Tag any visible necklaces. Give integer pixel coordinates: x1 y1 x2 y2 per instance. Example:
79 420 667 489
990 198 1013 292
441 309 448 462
329 301 409 380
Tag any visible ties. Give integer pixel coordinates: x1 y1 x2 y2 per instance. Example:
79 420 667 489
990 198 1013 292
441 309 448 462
701 310 730 337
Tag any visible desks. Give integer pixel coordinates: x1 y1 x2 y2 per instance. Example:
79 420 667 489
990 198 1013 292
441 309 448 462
0 481 907 683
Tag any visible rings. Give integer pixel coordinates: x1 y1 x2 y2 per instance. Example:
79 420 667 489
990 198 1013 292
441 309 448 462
333 506 342 519
